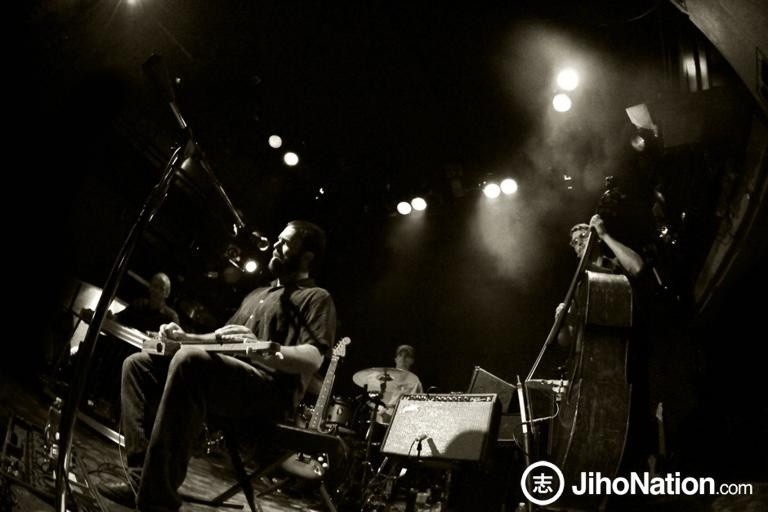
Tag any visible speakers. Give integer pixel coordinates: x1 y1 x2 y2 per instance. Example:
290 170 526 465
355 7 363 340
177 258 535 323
497 414 528 443
468 366 519 411
383 394 497 469
273 423 339 476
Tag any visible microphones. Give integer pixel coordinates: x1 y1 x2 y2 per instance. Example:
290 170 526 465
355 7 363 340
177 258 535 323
251 231 270 250
416 435 426 442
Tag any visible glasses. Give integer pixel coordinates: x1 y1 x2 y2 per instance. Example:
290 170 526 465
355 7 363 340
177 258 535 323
569 231 589 247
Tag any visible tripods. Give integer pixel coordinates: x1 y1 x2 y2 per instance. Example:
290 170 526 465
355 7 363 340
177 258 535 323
335 397 399 511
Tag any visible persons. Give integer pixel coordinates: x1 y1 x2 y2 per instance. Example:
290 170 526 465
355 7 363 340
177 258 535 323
554 214 644 348
367 346 425 467
97 219 336 512
93 271 181 421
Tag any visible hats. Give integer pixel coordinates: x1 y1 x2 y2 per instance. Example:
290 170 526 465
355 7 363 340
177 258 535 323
397 345 411 356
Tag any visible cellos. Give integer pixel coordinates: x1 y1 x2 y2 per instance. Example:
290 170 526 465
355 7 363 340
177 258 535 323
537 175 633 510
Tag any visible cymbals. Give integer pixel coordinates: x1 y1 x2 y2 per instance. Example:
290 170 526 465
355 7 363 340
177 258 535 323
352 368 416 390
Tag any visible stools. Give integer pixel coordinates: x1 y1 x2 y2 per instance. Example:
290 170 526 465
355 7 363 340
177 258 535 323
209 420 343 511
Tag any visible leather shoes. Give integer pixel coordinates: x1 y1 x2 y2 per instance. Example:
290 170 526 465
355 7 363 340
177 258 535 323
98 480 136 505
